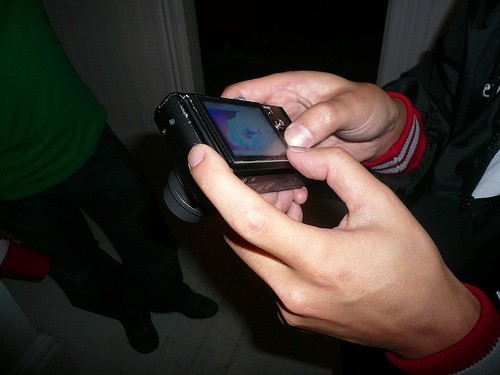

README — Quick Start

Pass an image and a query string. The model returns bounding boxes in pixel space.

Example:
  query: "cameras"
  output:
[154,91,313,223]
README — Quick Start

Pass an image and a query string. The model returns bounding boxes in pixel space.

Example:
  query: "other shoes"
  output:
[125,306,159,355]
[172,284,218,320]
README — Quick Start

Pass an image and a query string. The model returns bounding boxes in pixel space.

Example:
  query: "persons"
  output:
[0,0,217,356]
[187,0,500,375]
